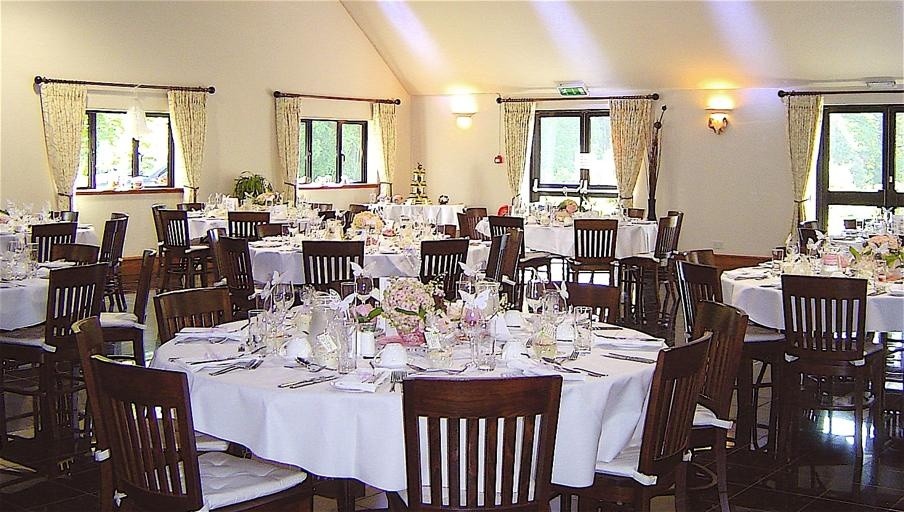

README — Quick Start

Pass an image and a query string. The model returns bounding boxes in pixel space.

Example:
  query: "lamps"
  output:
[705,107,734,133]
[452,112,474,129]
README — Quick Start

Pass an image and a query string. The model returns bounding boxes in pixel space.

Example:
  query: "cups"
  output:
[1,201,53,281]
[507,199,629,224]
[182,192,470,256]
[760,219,904,291]
[167,272,660,390]
